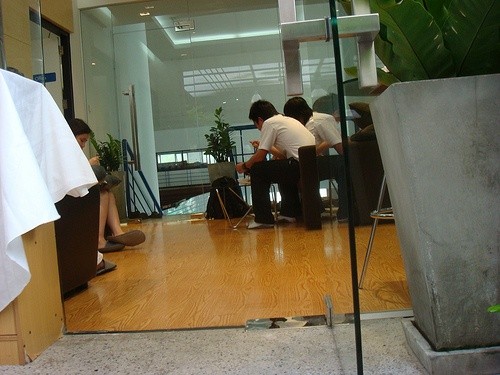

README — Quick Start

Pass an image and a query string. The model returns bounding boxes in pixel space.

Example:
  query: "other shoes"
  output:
[246,220,274,229]
[274,216,296,222]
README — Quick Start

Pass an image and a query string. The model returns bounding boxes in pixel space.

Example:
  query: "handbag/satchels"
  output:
[206,177,249,219]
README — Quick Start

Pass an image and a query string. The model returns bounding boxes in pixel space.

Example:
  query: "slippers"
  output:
[95,258,117,276]
[98,240,124,253]
[107,230,145,246]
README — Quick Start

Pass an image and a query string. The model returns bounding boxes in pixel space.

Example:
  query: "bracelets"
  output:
[242,161,250,172]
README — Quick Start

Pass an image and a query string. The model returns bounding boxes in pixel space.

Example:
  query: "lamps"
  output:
[250,88,261,103]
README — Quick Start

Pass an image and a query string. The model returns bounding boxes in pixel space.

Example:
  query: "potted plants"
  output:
[89,128,125,219]
[366,0,500,349]
[203,106,237,188]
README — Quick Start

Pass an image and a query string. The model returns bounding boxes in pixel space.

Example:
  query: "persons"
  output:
[235,94,360,229]
[67,119,146,275]
